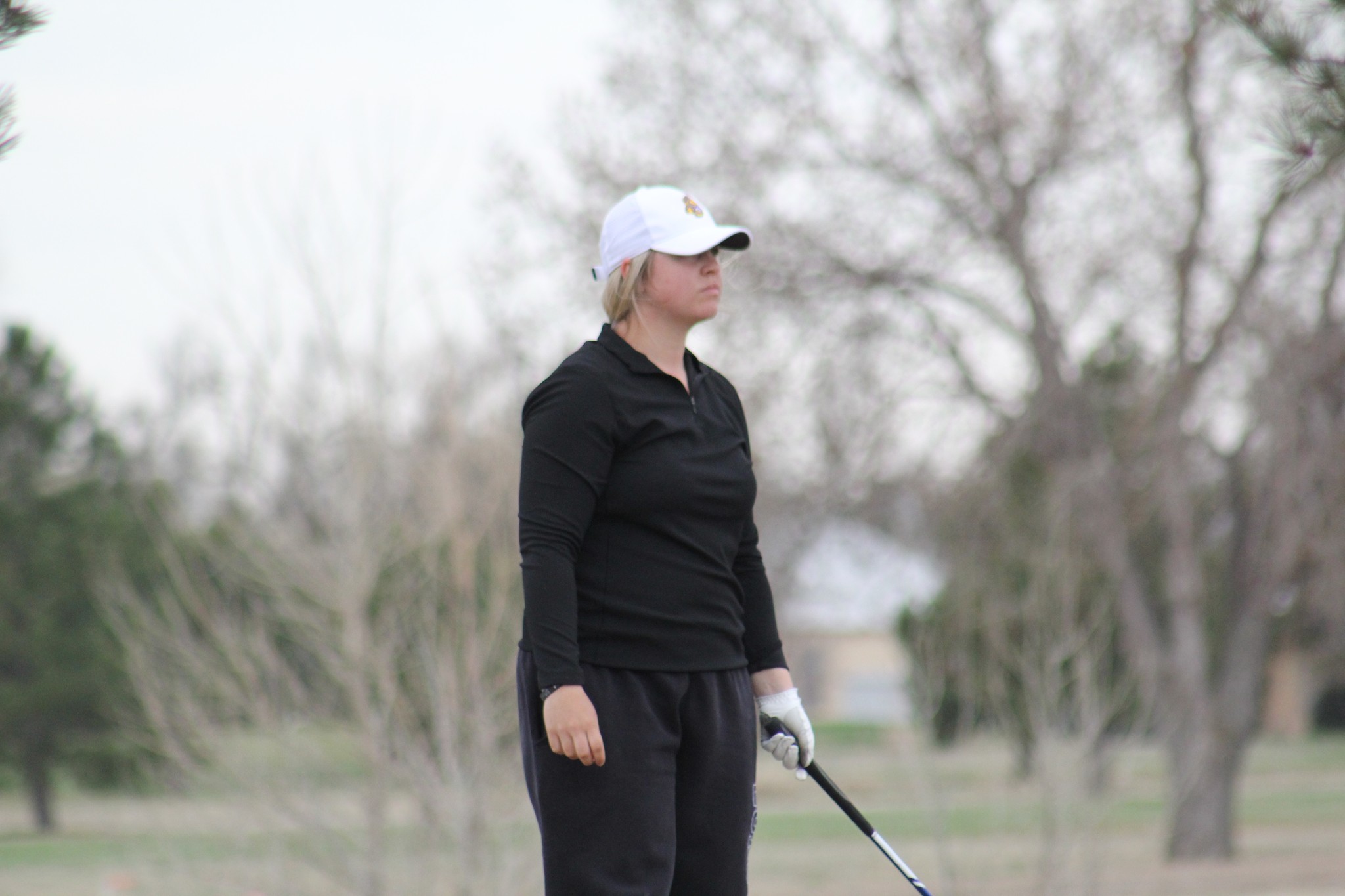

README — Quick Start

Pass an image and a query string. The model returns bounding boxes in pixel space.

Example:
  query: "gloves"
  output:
[756,688,815,779]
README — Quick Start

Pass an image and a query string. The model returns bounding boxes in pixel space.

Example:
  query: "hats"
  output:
[591,184,752,279]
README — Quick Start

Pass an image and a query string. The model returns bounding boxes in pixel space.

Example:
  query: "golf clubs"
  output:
[763,715,930,896]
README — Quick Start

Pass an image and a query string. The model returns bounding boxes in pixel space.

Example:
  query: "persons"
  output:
[516,186,815,896]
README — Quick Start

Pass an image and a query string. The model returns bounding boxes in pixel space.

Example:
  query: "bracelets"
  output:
[540,684,562,699]
